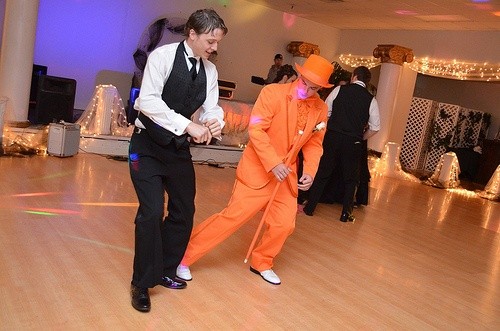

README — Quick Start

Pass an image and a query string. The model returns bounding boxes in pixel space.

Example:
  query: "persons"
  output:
[299,66,380,222]
[128,9,229,313]
[176,53,335,285]
[265,53,297,85]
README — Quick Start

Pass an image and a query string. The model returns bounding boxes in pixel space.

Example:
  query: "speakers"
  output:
[47,123,81,157]
[35,75,76,123]
[30,64,47,101]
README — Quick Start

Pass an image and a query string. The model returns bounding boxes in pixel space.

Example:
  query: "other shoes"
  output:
[250,265,281,286]
[303,206,314,216]
[340,212,356,223]
[176,264,193,281]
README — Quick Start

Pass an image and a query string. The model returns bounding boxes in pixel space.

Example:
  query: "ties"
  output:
[184,49,197,81]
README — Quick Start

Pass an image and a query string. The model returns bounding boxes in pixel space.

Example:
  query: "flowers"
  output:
[313,122,326,132]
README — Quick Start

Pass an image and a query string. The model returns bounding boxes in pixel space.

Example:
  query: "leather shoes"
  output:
[130,281,151,312]
[158,275,187,289]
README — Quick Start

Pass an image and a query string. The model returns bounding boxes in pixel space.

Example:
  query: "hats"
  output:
[275,54,283,59]
[295,54,334,88]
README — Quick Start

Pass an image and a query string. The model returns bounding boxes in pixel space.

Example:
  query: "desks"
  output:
[218,99,254,135]
